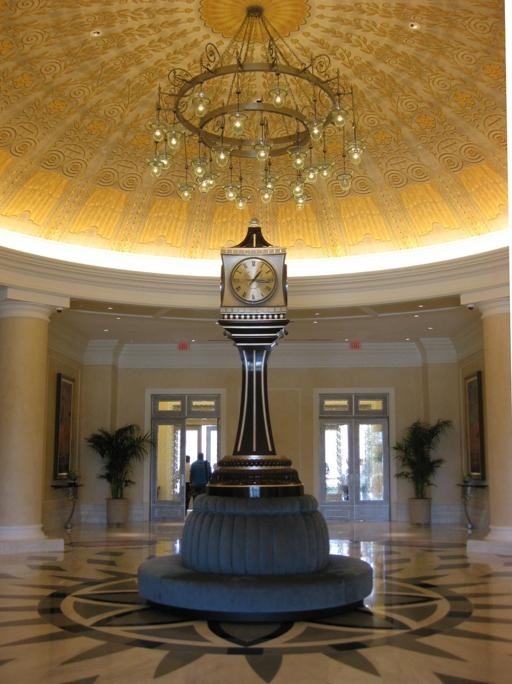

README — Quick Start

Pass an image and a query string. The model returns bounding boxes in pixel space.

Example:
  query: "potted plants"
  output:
[389,416,456,525]
[82,423,156,528]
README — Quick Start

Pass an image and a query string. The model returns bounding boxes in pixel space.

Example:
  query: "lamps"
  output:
[147,5,367,211]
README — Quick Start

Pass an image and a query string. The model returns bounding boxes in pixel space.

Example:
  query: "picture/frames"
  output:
[52,373,76,481]
[463,371,486,481]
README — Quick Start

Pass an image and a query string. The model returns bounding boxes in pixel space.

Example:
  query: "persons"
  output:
[184,455,194,517]
[189,453,212,500]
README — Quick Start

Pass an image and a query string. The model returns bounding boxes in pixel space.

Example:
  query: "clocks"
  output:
[204,215,304,498]
